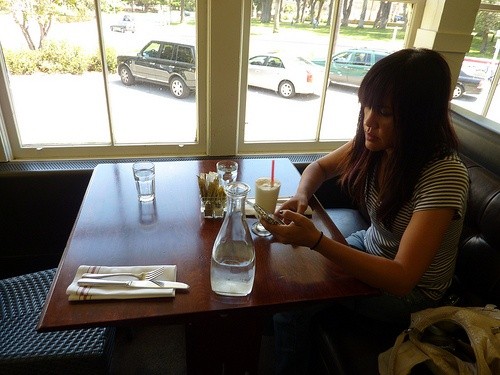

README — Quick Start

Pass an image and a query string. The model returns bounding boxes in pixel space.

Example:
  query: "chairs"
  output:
[0,269,115,375]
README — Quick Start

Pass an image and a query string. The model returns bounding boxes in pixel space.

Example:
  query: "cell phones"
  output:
[253,202,287,225]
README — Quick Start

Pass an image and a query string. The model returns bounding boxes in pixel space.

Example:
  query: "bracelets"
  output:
[310,231,323,249]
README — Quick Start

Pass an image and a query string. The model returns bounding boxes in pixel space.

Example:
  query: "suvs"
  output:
[116,38,197,99]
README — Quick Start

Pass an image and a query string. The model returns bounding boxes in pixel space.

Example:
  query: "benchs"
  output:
[320,153,500,375]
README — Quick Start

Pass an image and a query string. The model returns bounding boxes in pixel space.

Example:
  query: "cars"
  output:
[109,22,136,34]
[123,14,130,21]
[449,70,483,99]
[184,11,191,16]
[247,51,330,98]
[310,46,394,89]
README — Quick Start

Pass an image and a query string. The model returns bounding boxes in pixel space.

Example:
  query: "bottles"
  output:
[209,181,256,296]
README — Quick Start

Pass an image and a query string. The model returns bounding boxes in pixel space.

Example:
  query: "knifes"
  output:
[77,278,190,290]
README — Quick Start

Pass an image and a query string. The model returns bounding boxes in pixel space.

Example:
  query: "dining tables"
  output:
[35,157,385,375]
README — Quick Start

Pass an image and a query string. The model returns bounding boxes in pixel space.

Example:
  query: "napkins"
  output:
[66,265,178,301]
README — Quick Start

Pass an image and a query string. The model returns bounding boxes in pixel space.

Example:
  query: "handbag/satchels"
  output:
[387,305,500,375]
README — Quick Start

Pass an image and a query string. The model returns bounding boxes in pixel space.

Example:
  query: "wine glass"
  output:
[250,177,282,237]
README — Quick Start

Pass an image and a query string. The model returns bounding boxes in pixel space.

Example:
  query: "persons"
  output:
[260,48,470,375]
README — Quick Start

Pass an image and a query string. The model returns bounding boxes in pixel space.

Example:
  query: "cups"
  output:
[216,160,241,188]
[132,160,155,202]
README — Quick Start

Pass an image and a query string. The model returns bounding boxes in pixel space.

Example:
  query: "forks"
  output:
[82,267,168,281]
